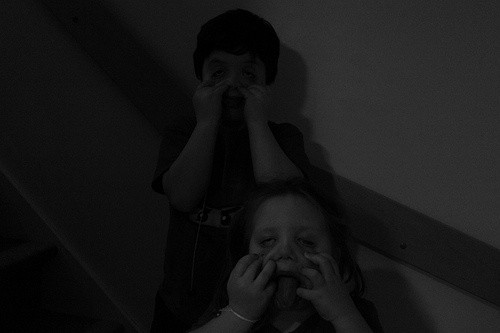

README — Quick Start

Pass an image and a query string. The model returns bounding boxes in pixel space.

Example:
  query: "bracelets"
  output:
[214,305,263,325]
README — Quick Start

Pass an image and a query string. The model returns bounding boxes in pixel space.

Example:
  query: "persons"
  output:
[146,5,313,333]
[184,176,386,333]
